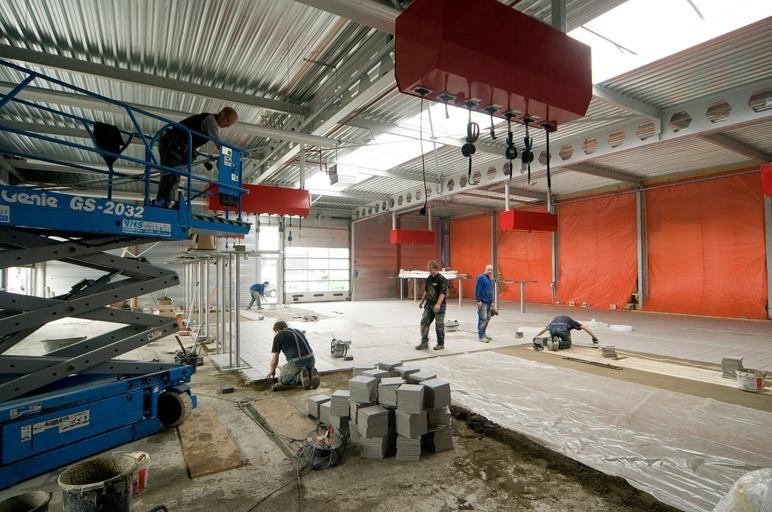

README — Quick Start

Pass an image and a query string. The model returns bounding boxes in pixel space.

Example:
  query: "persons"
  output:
[533,316,599,351]
[267,322,320,390]
[416,261,447,350]
[475,265,495,343]
[247,282,269,309]
[157,107,238,208]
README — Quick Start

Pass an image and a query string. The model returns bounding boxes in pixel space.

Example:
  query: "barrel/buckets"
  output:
[57,454,147,512]
[0,490,53,512]
[128,450,150,501]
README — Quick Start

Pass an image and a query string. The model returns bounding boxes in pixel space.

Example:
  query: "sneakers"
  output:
[434,344,444,350]
[546,336,559,351]
[311,368,320,389]
[481,336,492,343]
[416,343,428,349]
[300,368,311,389]
